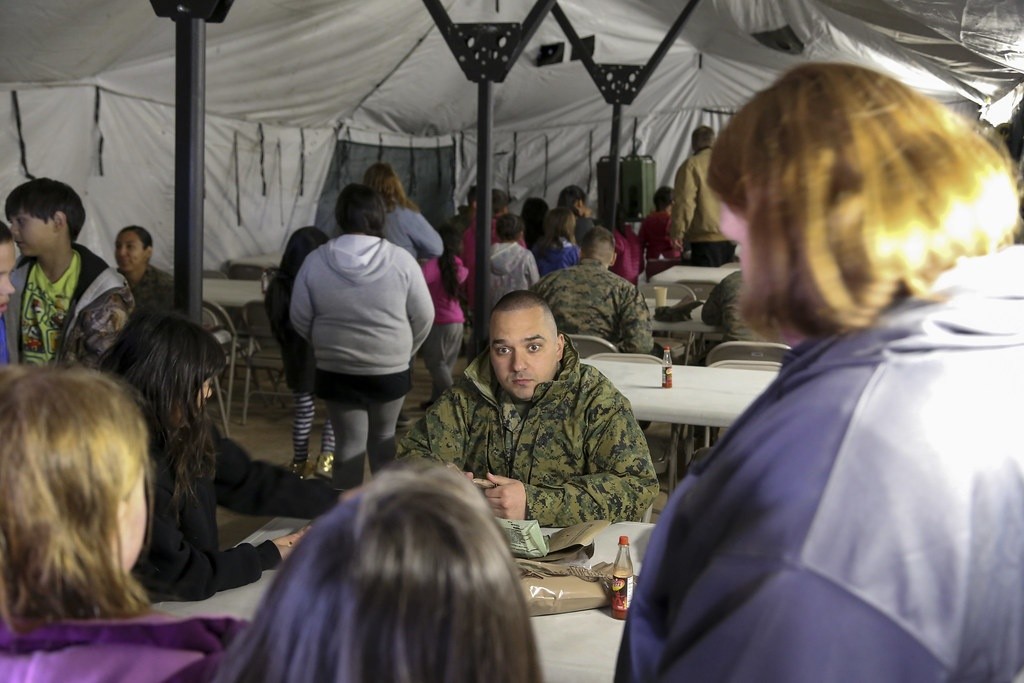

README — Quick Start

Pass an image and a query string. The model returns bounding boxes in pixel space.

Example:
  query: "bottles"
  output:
[611,536,633,618]
[662,346,672,388]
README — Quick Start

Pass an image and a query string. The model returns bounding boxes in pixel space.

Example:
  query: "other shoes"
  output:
[285,455,314,481]
[313,453,335,482]
[396,412,411,426]
[420,401,434,411]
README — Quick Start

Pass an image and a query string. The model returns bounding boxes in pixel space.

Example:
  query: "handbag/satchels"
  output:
[513,558,641,617]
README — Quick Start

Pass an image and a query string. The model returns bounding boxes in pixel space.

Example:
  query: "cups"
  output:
[653,287,667,307]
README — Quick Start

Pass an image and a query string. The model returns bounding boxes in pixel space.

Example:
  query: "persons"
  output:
[395,291,658,531]
[216,462,539,683]
[264,124,788,485]
[0,179,361,683]
[611,64,1024,683]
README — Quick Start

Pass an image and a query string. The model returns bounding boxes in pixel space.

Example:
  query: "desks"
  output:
[202,279,271,310]
[579,357,782,427]
[145,515,657,683]
[649,266,741,284]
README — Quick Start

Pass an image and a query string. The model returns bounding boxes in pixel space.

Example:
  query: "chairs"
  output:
[584,277,792,501]
[201,264,284,442]
